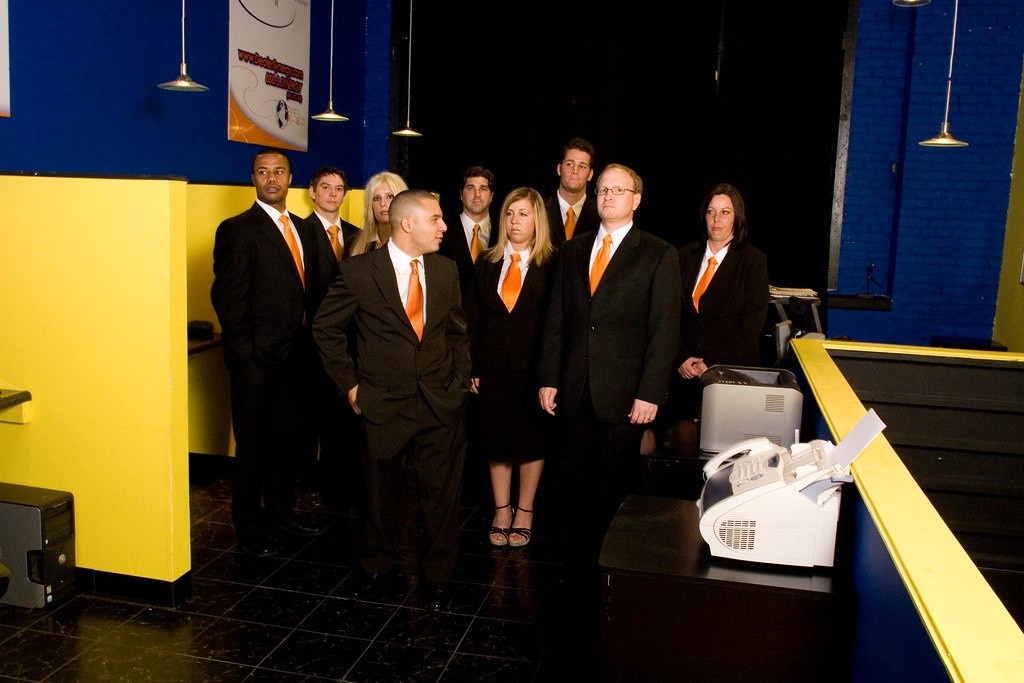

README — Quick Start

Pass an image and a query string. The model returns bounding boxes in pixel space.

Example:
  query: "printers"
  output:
[697,408,887,566]
[698,364,802,461]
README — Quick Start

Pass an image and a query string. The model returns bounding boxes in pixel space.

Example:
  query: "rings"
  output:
[644,417,650,420]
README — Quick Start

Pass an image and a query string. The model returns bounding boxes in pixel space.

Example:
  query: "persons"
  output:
[350,170,410,549]
[435,166,501,293]
[675,183,770,494]
[537,163,682,474]
[311,189,473,573]
[211,147,318,557]
[540,137,602,252]
[304,165,366,382]
[460,188,560,546]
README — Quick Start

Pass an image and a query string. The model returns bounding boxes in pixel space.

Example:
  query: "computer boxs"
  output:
[0,482,76,609]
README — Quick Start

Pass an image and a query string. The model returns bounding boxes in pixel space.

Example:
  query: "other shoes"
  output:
[240,533,276,557]
[292,506,329,532]
[426,581,450,613]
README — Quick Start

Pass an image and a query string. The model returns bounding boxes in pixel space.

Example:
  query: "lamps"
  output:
[311,0,349,122]
[392,0,423,136]
[918,0,969,147]
[157,0,210,92]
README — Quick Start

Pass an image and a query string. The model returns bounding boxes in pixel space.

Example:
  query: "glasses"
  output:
[595,186,638,195]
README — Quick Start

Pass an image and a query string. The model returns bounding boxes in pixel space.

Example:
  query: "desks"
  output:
[597,420,833,683]
[769,295,823,334]
[189,332,222,356]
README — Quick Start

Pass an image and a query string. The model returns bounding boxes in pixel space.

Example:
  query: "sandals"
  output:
[489,503,515,546]
[509,506,535,549]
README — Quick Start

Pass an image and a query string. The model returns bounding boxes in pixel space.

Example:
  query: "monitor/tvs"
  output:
[774,296,822,362]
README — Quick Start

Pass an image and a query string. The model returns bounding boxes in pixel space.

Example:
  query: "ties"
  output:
[327,226,344,264]
[501,255,522,314]
[470,224,482,265]
[589,235,613,297]
[278,215,307,324]
[564,207,578,241]
[404,259,424,343]
[692,257,718,313]
